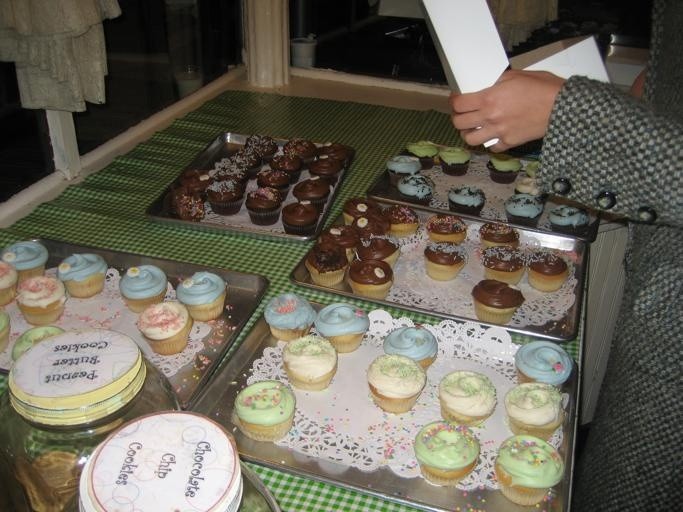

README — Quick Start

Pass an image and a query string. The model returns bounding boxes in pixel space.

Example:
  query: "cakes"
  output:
[227,379,297,444]
[514,338,575,389]
[304,196,570,326]
[1,240,228,363]
[502,380,565,441]
[493,433,566,509]
[385,139,592,236]
[413,418,483,487]
[163,132,348,237]
[439,369,497,432]
[263,291,440,416]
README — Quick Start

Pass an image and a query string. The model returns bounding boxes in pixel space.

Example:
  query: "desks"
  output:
[0,65,597,507]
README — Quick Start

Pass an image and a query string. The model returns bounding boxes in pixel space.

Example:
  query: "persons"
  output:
[448,2,680,512]
